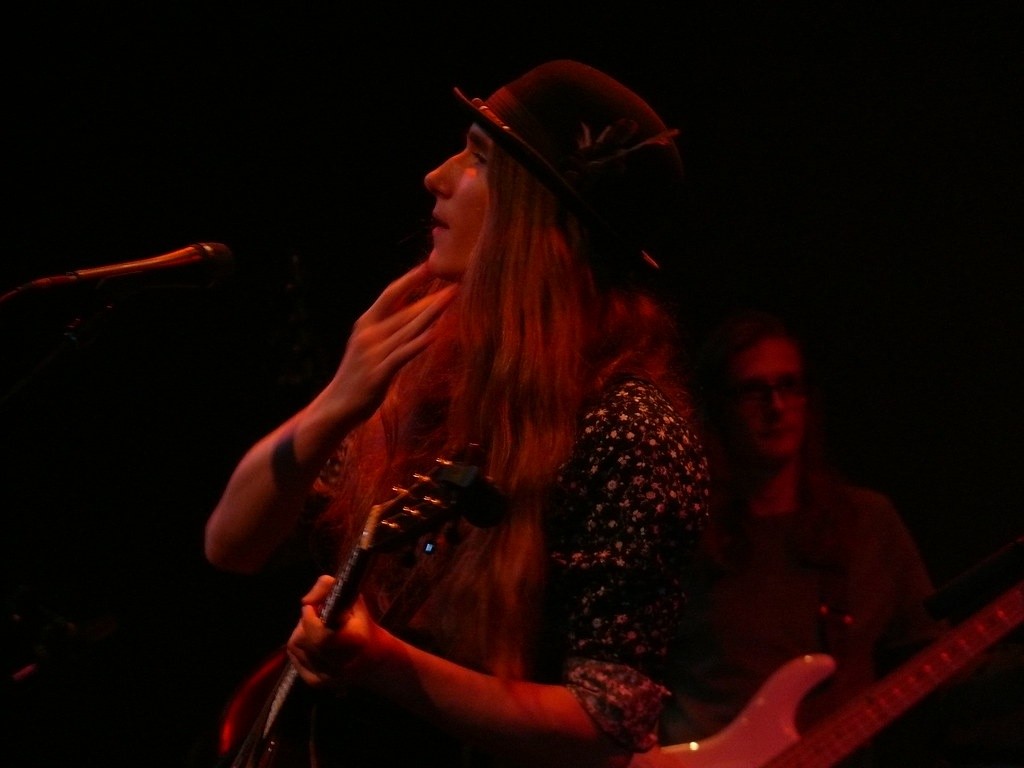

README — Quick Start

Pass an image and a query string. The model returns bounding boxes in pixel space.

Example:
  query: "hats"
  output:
[453,59,687,290]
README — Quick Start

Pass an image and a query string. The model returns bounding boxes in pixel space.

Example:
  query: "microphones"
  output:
[24,243,237,298]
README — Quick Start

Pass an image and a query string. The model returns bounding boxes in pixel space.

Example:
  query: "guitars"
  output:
[232,444,493,768]
[629,582,1023,766]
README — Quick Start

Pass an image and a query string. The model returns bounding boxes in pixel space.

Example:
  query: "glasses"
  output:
[709,368,819,408]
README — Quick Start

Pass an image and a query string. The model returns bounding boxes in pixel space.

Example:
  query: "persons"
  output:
[697,315,1001,763]
[206,61,707,768]
